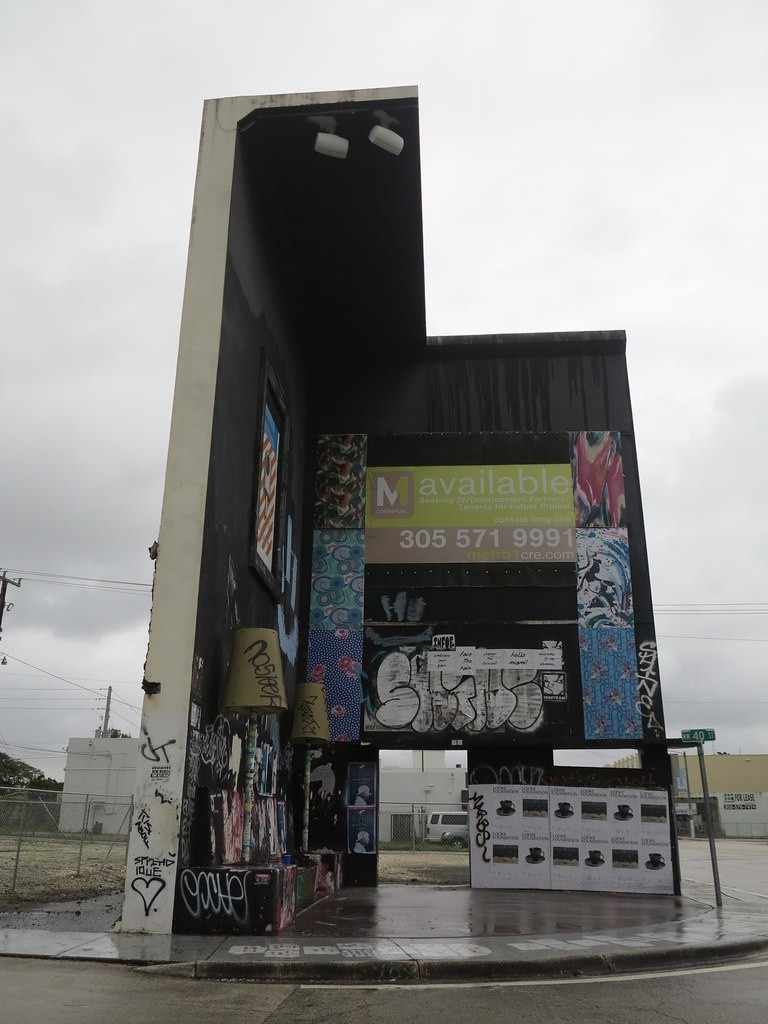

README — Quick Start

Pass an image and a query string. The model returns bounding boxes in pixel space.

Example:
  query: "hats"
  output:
[355,831,369,841]
[356,785,369,795]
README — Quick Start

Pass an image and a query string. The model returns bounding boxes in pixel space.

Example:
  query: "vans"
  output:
[424,811,469,849]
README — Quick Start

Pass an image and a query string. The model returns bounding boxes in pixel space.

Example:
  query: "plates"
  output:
[613,812,634,821]
[496,807,516,817]
[584,857,605,868]
[554,810,575,818]
[617,804,633,818]
[525,855,546,864]
[644,861,666,870]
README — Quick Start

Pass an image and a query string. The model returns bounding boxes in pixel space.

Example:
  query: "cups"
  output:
[648,853,665,867]
[500,800,515,813]
[528,847,545,861]
[558,802,573,815]
[588,849,604,865]
[649,853,664,866]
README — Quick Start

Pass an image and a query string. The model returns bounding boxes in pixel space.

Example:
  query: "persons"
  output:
[355,785,370,806]
[354,831,369,853]
